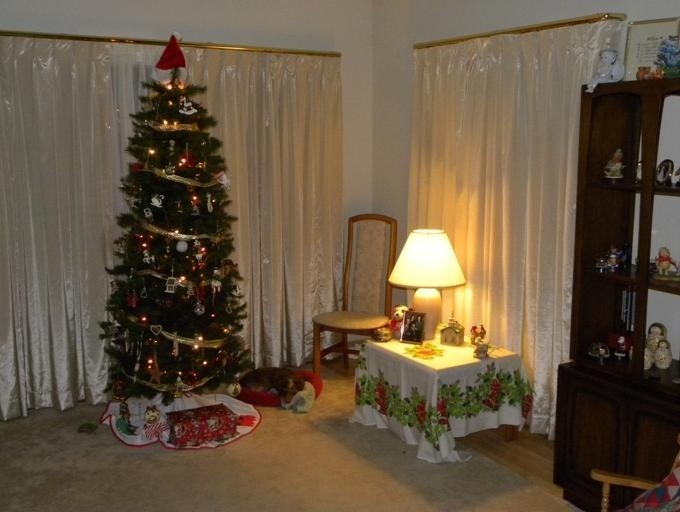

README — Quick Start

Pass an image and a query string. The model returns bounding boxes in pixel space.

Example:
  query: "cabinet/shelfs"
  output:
[551,76,680,404]
[550,359,680,512]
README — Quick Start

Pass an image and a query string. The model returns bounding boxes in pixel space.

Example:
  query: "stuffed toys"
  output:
[384,305,414,343]
[655,247,679,276]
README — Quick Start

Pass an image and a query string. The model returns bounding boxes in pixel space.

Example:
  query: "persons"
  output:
[404,313,423,342]
[584,43,618,93]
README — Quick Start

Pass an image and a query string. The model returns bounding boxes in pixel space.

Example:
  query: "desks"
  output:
[347,336,535,464]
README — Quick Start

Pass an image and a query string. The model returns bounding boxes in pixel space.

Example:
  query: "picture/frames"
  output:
[623,17,679,79]
[401,309,427,345]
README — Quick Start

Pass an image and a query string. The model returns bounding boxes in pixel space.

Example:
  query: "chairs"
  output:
[312,212,398,379]
[588,431,680,512]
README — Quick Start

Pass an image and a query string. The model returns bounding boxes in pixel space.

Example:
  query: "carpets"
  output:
[0,358,587,511]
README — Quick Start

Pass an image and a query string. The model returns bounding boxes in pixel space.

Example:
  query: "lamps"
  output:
[387,228,468,342]
